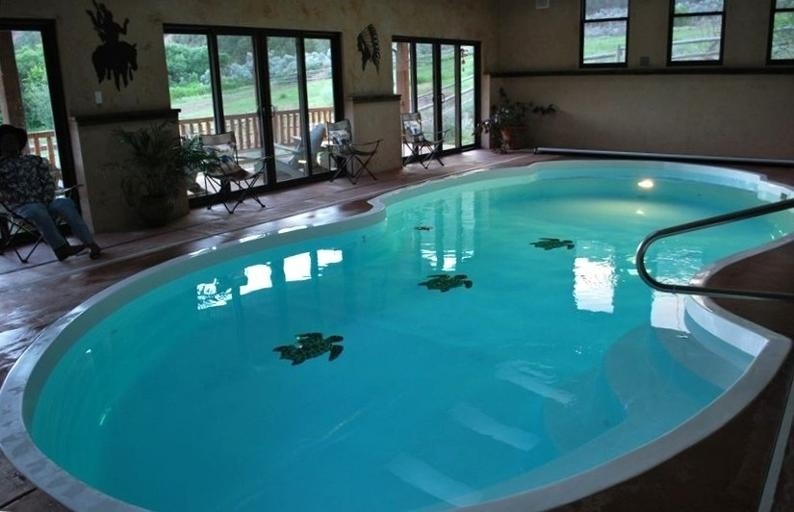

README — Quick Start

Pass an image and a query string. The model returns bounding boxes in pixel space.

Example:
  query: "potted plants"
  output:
[107,115,231,229]
[472,83,559,149]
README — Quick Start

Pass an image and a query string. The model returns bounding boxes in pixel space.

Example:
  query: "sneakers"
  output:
[54,242,88,262]
[84,241,101,260]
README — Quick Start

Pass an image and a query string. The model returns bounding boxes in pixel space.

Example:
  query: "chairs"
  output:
[253,117,384,186]
[200,130,269,214]
[0,160,85,265]
[399,111,450,171]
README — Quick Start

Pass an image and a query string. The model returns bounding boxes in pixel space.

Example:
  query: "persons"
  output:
[0,124,101,261]
